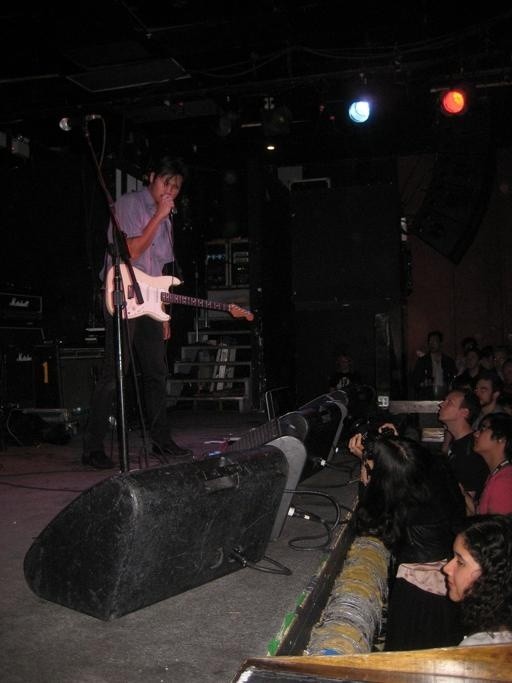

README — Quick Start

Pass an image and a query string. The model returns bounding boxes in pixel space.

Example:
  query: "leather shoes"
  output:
[152,440,192,459]
[81,452,115,469]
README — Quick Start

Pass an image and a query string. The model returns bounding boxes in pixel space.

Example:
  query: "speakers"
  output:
[24,444,289,622]
[229,389,349,539]
[40,343,115,422]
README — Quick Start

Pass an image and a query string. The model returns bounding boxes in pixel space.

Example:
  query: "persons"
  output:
[328,332,511,652]
[82,148,193,470]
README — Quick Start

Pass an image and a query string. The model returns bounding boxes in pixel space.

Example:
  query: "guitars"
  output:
[105,264,254,322]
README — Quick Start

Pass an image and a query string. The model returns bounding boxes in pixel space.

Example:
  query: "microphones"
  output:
[59,114,98,132]
[168,203,177,216]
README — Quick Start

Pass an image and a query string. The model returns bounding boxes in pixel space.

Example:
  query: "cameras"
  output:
[351,419,395,455]
